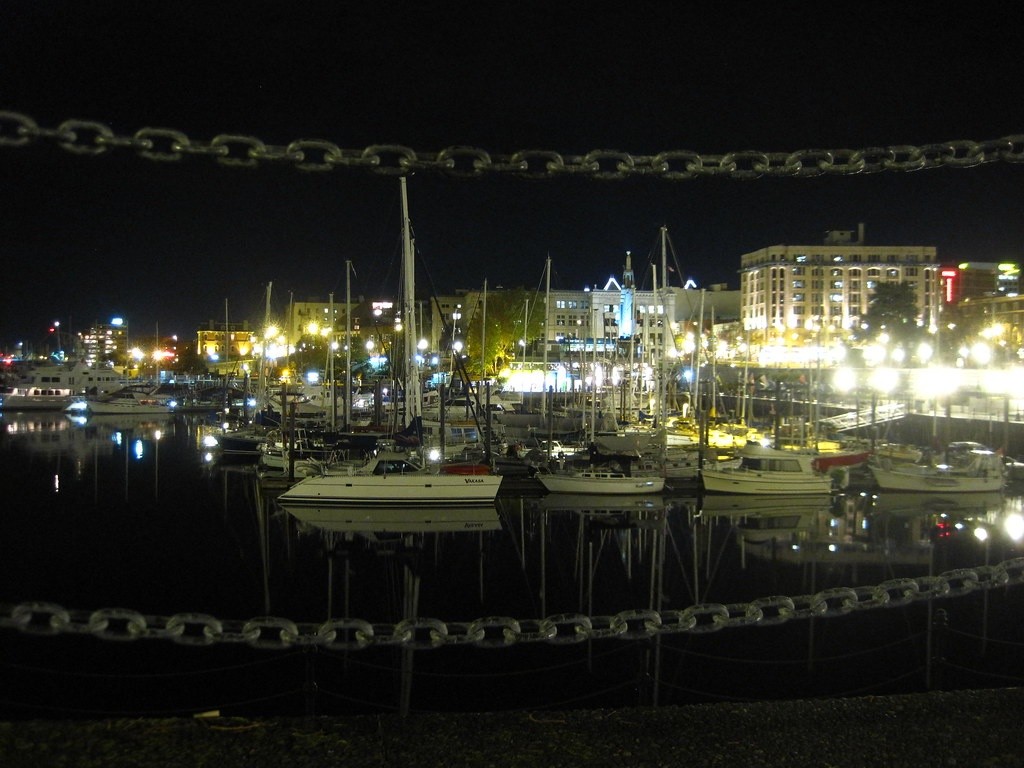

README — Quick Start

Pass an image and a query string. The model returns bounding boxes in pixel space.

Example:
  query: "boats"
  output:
[700,443,834,497]
[2,321,128,410]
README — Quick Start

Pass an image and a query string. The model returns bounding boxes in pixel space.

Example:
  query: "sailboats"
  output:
[512,221,706,497]
[217,261,507,478]
[277,504,504,715]
[86,321,175,415]
[276,174,506,502]
[864,258,1024,493]
[524,492,842,710]
[516,252,870,480]
